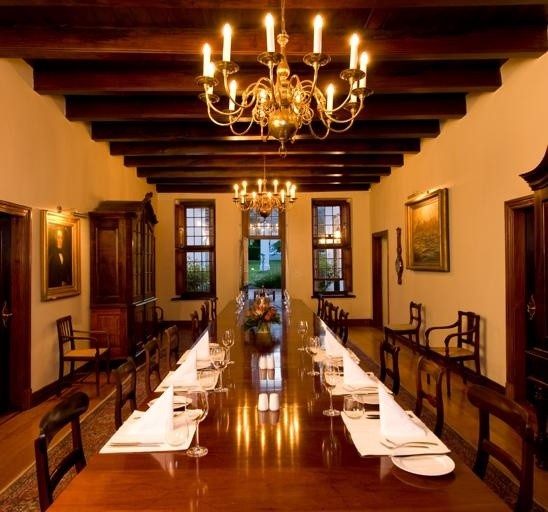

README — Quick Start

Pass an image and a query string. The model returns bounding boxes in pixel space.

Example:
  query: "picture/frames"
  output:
[40,208,81,301]
[404,188,450,272]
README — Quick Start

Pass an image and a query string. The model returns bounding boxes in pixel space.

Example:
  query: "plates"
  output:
[391,454,457,476]
[149,395,190,409]
[355,392,391,404]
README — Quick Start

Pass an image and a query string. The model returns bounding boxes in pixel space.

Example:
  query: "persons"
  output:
[49,227,68,288]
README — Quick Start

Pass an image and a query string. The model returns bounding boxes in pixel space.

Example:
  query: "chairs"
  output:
[34,393,89,512]
[467,385,538,512]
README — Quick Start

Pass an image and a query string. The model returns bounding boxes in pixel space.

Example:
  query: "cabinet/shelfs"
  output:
[89,192,158,359]
[503,145,548,470]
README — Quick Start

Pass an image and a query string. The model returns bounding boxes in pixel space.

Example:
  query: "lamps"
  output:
[193,0,374,158]
[232,156,299,223]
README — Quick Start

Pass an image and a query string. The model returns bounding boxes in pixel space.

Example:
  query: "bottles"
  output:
[258,393,267,413]
[268,394,280,414]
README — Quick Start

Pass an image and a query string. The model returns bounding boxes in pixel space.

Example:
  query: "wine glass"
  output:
[186,455,208,511]
[184,288,365,459]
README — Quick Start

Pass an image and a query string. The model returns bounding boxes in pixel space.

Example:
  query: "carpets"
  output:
[0,334,548,512]
